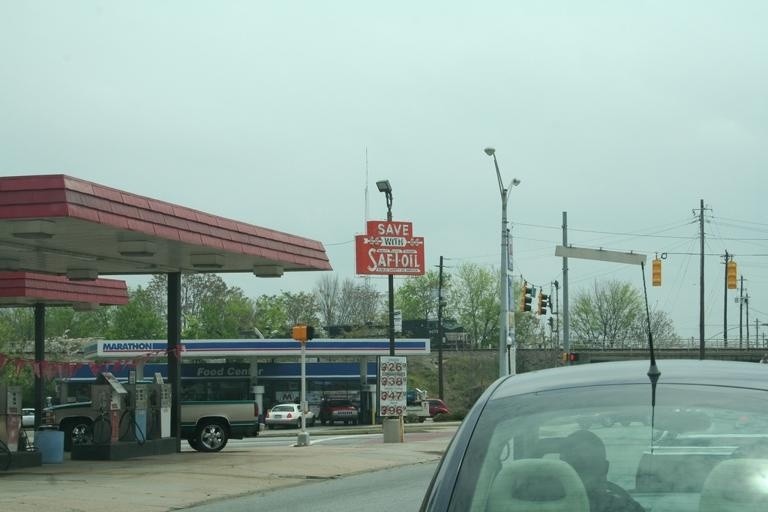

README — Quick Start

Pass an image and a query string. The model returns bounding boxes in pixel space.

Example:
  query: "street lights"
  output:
[484,147,521,379]
[375,180,405,442]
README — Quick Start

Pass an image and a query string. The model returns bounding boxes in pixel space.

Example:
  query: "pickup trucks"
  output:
[38,380,260,453]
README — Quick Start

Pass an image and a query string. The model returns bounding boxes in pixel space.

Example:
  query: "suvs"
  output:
[424,398,450,421]
[318,400,359,425]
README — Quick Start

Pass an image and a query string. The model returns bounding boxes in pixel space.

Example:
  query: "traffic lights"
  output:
[293,325,315,341]
[546,316,553,327]
[546,294,553,308]
[570,353,579,360]
[518,284,533,313]
[537,292,547,315]
[562,352,569,362]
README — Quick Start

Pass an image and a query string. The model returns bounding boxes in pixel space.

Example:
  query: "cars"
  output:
[265,402,316,430]
[419,261,768,511]
[575,404,651,428]
[20,407,35,428]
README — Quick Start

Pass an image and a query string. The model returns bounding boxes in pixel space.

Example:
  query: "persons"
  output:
[51,394,61,406]
[559,429,645,511]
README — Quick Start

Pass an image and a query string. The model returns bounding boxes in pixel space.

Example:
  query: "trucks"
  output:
[403,388,430,422]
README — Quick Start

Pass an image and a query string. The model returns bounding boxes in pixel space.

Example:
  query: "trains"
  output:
[183,317,472,351]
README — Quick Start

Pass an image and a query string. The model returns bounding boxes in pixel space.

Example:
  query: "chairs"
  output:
[486,458,589,511]
[697,456,766,511]
